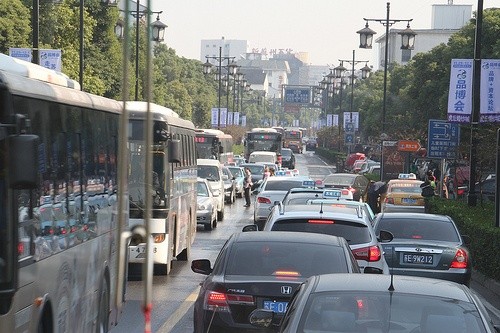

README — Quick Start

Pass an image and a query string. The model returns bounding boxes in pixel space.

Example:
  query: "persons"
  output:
[243,167,252,207]
[205,169,216,178]
[417,162,448,201]
[263,166,275,179]
[127,155,159,205]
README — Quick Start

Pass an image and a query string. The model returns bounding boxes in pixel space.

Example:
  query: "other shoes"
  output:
[243,204,250,207]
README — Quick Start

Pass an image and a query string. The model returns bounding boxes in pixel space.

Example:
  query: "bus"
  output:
[194,128,234,163]
[273,126,303,154]
[242,128,282,165]
[117,101,197,275]
[0,52,129,333]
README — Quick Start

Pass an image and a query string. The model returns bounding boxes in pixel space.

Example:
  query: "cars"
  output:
[15,179,117,260]
[352,160,381,175]
[281,148,296,168]
[251,173,375,231]
[371,212,472,284]
[302,137,319,151]
[377,179,425,213]
[248,271,500,333]
[191,231,383,333]
[181,151,280,231]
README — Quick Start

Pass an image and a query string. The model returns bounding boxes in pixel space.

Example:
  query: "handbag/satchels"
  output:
[249,182,253,186]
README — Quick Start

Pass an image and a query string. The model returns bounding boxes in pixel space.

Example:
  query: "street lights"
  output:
[119,11,169,101]
[201,56,250,129]
[356,18,417,181]
[317,60,372,154]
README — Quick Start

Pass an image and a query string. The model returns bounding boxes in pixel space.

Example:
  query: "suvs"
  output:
[241,201,393,276]
